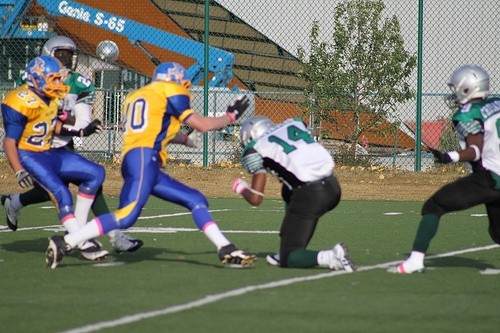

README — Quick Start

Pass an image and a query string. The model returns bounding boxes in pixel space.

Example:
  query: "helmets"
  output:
[41,36,78,71]
[238,116,275,149]
[152,62,191,89]
[26,56,70,99]
[444,65,489,108]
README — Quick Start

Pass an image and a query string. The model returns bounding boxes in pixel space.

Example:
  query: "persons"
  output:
[0,35,144,254]
[232,115,356,271]
[386,64,500,273]
[0,56,110,262]
[44,61,257,270]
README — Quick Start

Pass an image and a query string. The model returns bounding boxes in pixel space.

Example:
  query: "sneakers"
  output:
[386,262,425,273]
[266,253,280,265]
[78,239,101,251]
[329,242,357,272]
[81,249,109,261]
[110,234,144,254]
[218,247,258,266]
[45,235,72,269]
[0,194,19,231]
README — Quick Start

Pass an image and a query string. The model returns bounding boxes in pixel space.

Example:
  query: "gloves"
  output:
[232,178,248,195]
[428,146,460,165]
[81,119,105,137]
[186,133,200,147]
[227,95,250,121]
[15,169,34,189]
